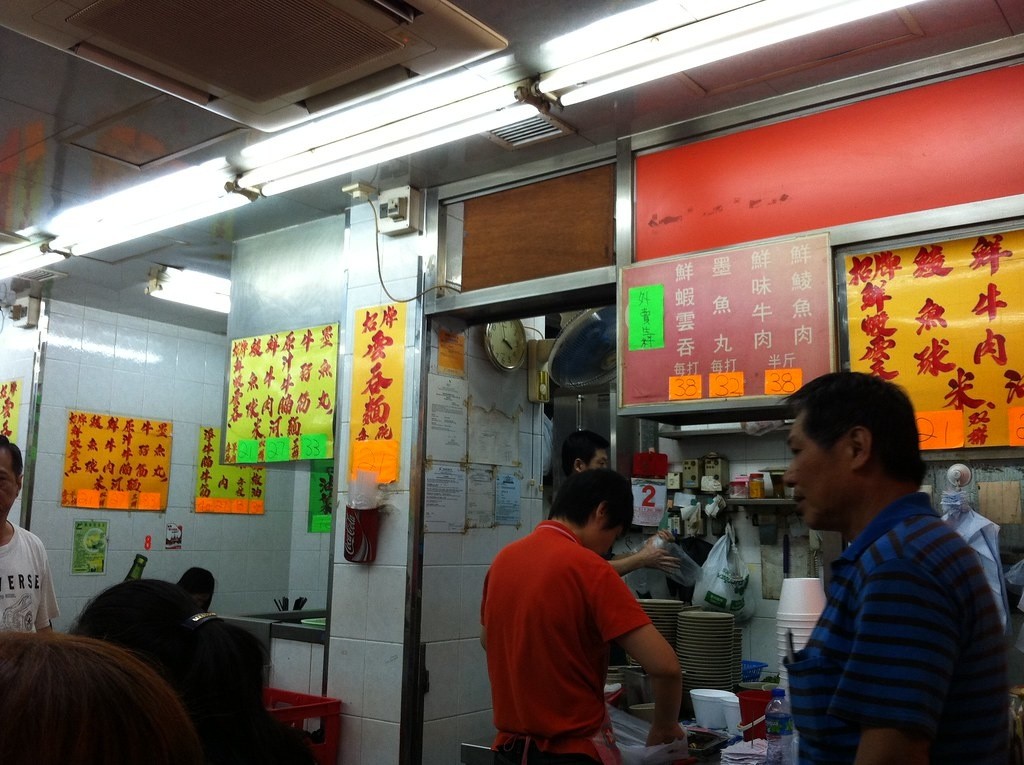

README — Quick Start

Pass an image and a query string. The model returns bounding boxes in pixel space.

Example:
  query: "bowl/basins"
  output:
[723,696,744,738]
[777,575,828,700]
[690,688,738,728]
[628,704,659,722]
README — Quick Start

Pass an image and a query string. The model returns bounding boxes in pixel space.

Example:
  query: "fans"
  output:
[525,304,618,492]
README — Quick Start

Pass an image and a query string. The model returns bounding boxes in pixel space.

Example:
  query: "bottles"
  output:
[729,479,750,499]
[120,554,148,584]
[769,469,786,500]
[749,472,764,499]
[765,687,793,765]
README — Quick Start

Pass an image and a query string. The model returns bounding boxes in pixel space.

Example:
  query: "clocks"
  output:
[483,316,529,374]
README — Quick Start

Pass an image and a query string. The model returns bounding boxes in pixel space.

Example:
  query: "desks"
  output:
[459,701,793,764]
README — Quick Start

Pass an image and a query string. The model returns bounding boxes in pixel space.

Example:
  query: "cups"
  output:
[344,503,383,564]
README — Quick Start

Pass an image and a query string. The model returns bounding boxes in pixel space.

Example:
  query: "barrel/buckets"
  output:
[736,691,781,742]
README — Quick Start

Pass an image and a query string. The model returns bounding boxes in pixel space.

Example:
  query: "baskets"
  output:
[741,659,769,682]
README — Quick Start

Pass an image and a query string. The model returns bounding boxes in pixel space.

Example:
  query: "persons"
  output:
[0,434,62,635]
[772,372,1013,765]
[0,567,320,765]
[478,468,686,765]
[548,430,682,578]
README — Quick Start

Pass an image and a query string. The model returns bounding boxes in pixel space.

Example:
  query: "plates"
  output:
[761,682,779,691]
[629,597,745,699]
[738,681,769,691]
[605,667,625,687]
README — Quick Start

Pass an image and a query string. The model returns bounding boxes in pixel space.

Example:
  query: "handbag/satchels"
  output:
[690,524,755,623]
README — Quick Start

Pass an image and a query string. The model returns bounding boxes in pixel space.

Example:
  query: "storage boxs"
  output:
[265,686,344,763]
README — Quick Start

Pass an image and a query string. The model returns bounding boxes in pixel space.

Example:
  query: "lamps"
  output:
[1,2,932,284]
